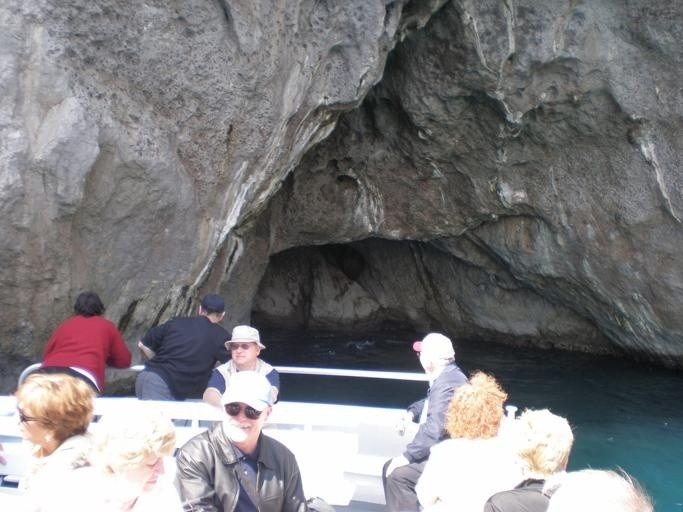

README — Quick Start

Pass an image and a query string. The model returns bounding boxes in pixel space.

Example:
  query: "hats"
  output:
[220,370,272,411]
[223,324,266,350]
[413,331,455,360]
[201,294,226,314]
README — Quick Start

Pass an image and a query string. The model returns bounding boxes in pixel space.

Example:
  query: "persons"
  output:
[75,398,183,512]
[38,291,132,398]
[483,408,574,512]
[542,466,655,512]
[203,325,280,408]
[15,372,100,512]
[134,294,232,401]
[415,370,517,512]
[382,332,469,512]
[175,371,307,512]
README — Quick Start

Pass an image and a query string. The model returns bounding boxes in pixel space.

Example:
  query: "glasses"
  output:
[16,408,44,423]
[221,400,263,420]
[228,342,257,350]
